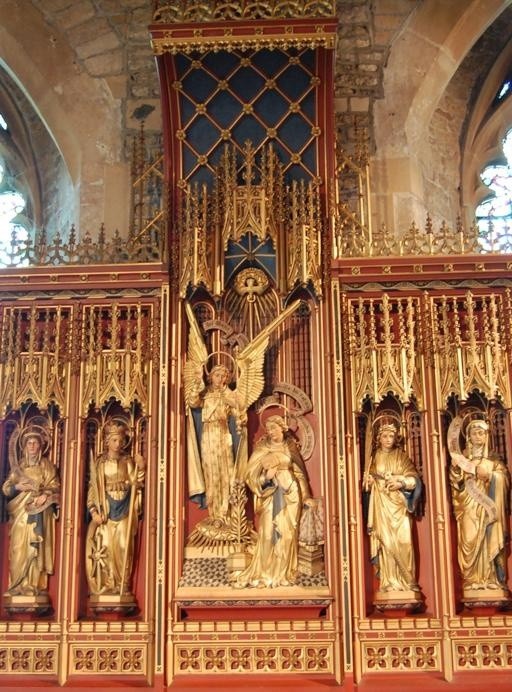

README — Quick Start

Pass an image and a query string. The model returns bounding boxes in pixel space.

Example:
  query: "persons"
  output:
[192,363,246,524]
[449,420,505,589]
[362,423,425,592]
[227,414,312,585]
[82,421,143,596]
[1,430,60,602]
